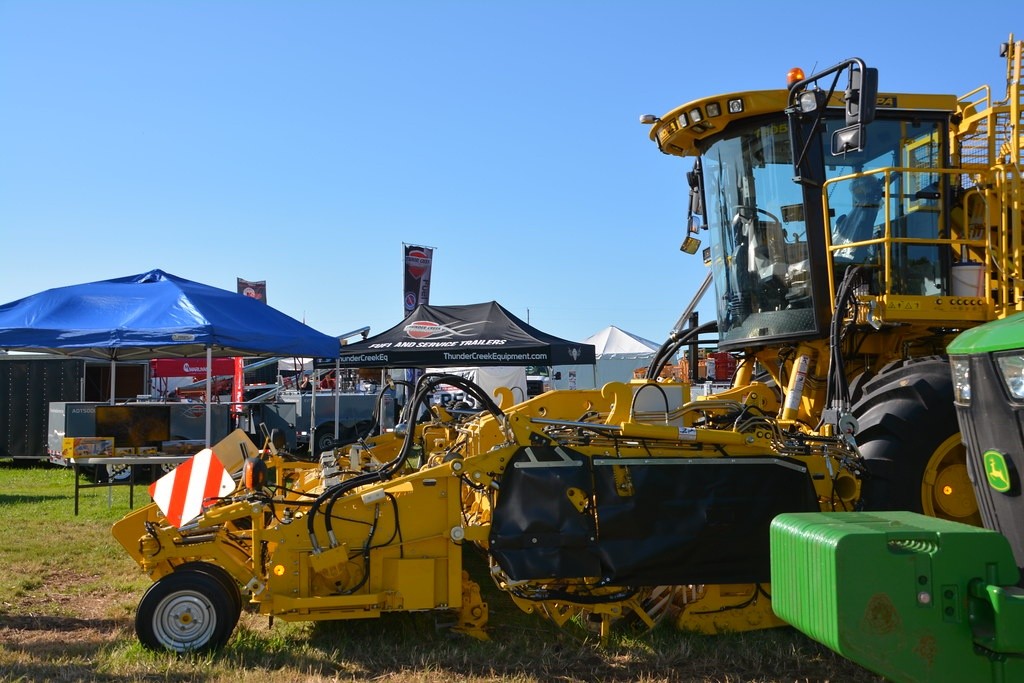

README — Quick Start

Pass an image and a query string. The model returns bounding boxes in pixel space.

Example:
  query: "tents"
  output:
[0,269,340,465]
[310,301,679,457]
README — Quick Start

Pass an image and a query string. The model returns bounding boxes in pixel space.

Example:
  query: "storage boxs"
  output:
[63,437,114,458]
[137,447,157,455]
[114,447,136,457]
[162,439,206,455]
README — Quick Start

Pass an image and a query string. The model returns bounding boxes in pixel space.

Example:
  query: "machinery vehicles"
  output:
[767,310,1024,683]
[109,33,1024,661]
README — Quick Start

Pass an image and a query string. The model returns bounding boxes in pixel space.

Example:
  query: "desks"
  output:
[71,455,194,516]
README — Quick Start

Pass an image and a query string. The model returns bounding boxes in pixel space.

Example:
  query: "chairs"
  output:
[788,175,883,284]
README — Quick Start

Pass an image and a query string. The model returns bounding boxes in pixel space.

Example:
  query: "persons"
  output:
[300,374,312,394]
[284,377,294,389]
[320,375,336,393]
